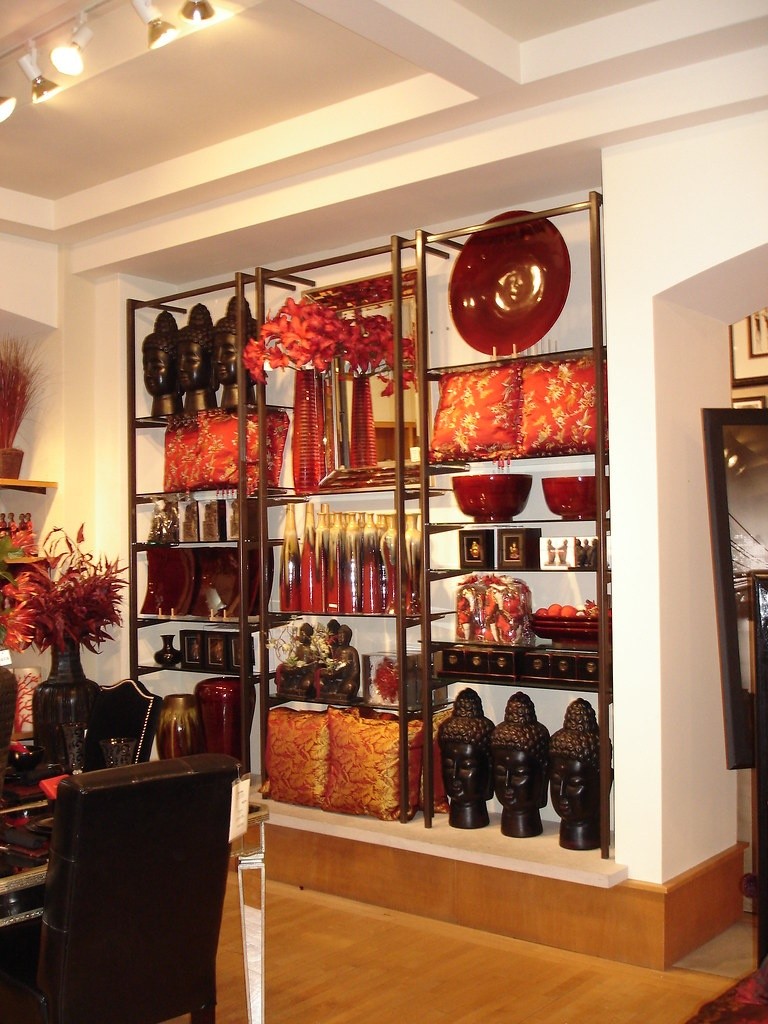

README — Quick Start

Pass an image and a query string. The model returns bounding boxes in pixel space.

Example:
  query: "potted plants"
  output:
[0,332,59,479]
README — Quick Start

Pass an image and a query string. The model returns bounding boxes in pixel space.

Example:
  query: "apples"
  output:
[536,604,578,617]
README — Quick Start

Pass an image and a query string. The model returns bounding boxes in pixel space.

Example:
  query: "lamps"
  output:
[0,96,16,123]
[17,39,61,104]
[50,12,94,75]
[130,0,177,49]
[181,0,214,21]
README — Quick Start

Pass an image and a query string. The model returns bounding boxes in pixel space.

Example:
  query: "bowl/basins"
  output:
[452,474,533,522]
[542,476,610,520]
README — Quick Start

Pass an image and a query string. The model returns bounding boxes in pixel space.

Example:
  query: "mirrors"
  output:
[701,407,768,772]
[301,265,434,494]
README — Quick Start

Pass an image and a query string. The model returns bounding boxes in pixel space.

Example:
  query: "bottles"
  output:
[155,635,180,665]
[279,503,423,614]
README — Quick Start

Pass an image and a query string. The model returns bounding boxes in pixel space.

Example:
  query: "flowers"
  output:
[241,296,364,387]
[345,307,418,398]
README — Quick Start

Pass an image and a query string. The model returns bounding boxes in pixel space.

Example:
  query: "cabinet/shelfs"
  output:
[0,478,58,563]
[126,191,616,861]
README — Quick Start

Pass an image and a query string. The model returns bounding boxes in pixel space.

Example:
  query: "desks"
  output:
[0,798,271,1024]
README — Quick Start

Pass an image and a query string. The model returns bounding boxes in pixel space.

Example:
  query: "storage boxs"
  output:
[361,647,448,710]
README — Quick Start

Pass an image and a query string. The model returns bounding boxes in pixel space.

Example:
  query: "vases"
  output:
[294,370,325,494]
[280,503,423,615]
[350,377,377,467]
[155,636,180,667]
[0,666,17,798]
[32,638,99,762]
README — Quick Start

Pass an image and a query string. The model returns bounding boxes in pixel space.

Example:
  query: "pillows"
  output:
[163,409,291,497]
[428,354,609,469]
[257,706,453,821]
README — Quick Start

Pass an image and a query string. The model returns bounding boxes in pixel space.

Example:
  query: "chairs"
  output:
[84,679,163,774]
[1,754,241,1024]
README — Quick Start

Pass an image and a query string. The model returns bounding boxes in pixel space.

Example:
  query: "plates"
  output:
[530,614,612,637]
[448,210,572,355]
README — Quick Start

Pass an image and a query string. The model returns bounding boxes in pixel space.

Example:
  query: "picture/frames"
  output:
[728,306,768,389]
[732,395,765,410]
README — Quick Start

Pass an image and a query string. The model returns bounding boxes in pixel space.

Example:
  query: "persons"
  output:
[437,687,614,851]
[275,619,360,696]
[142,296,257,417]
